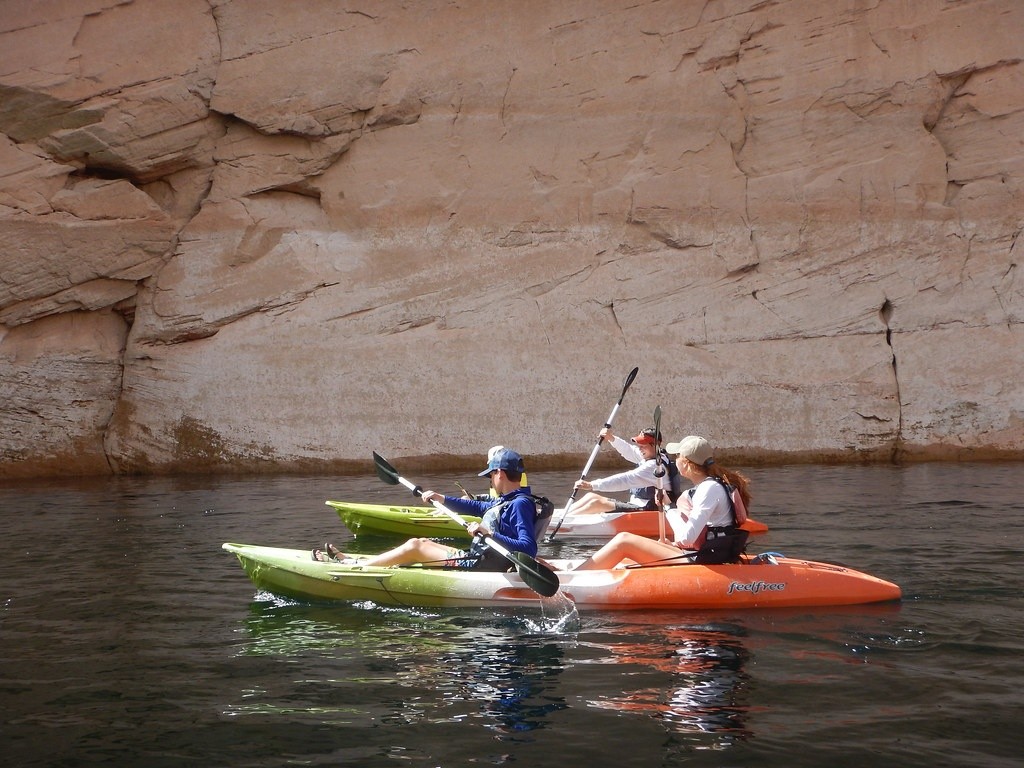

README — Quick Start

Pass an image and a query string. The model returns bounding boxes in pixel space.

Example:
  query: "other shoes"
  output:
[311,542,340,562]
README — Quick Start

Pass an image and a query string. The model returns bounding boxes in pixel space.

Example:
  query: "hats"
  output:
[631,427,662,445]
[486,445,504,464]
[478,449,525,476]
[666,436,714,465]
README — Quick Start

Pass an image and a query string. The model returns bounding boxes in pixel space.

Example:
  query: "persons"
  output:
[312,447,536,573]
[458,444,533,503]
[566,426,680,514]
[536,434,753,572]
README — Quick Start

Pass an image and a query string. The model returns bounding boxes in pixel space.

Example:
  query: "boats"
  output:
[219,542,904,612]
[326,500,771,542]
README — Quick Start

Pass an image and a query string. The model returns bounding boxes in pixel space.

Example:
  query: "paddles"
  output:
[372,449,560,599]
[652,404,670,543]
[546,365,640,542]
